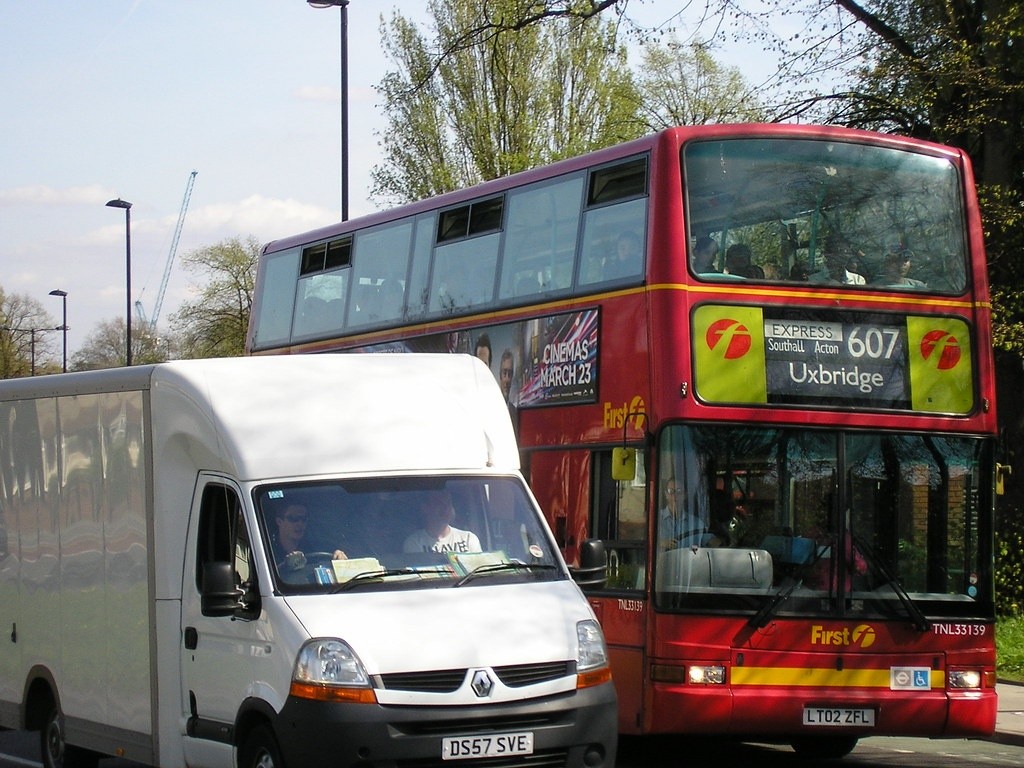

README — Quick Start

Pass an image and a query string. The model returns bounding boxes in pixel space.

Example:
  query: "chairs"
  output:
[235,507,251,581]
[300,276,539,333]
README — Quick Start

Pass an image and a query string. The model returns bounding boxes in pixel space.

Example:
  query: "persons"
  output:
[656,476,722,554]
[403,484,483,553]
[801,489,868,592]
[474,335,518,442]
[297,231,927,335]
[250,495,349,573]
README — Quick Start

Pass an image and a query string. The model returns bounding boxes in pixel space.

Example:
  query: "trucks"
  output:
[1,352,620,767]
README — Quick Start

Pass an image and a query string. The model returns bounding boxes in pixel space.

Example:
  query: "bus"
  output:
[243,123,1013,763]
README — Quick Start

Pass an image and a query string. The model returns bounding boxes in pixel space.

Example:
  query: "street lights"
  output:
[48,289,68,373]
[1,325,71,377]
[307,0,350,221]
[105,200,132,365]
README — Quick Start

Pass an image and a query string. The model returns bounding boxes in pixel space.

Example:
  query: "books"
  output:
[313,550,523,584]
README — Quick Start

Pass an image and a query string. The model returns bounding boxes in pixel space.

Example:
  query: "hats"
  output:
[884,243,915,258]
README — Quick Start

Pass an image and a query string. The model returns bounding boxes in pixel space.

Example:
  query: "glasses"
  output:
[666,487,682,495]
[281,515,309,522]
